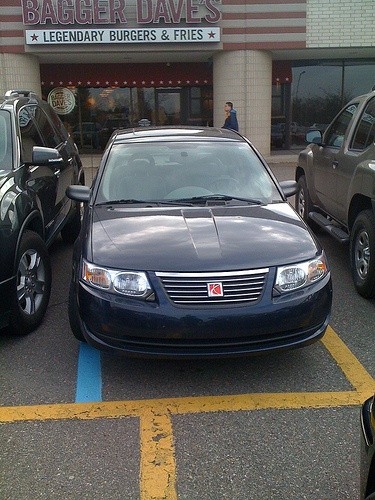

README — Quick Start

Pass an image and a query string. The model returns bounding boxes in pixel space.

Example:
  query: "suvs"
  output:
[0,88,85,335]
[294,86,375,300]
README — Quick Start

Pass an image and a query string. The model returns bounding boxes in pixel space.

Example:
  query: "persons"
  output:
[221,112,230,128]
[224,102,238,132]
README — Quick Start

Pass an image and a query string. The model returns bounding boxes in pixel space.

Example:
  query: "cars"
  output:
[271,125,284,149]
[72,122,100,146]
[65,125,334,362]
